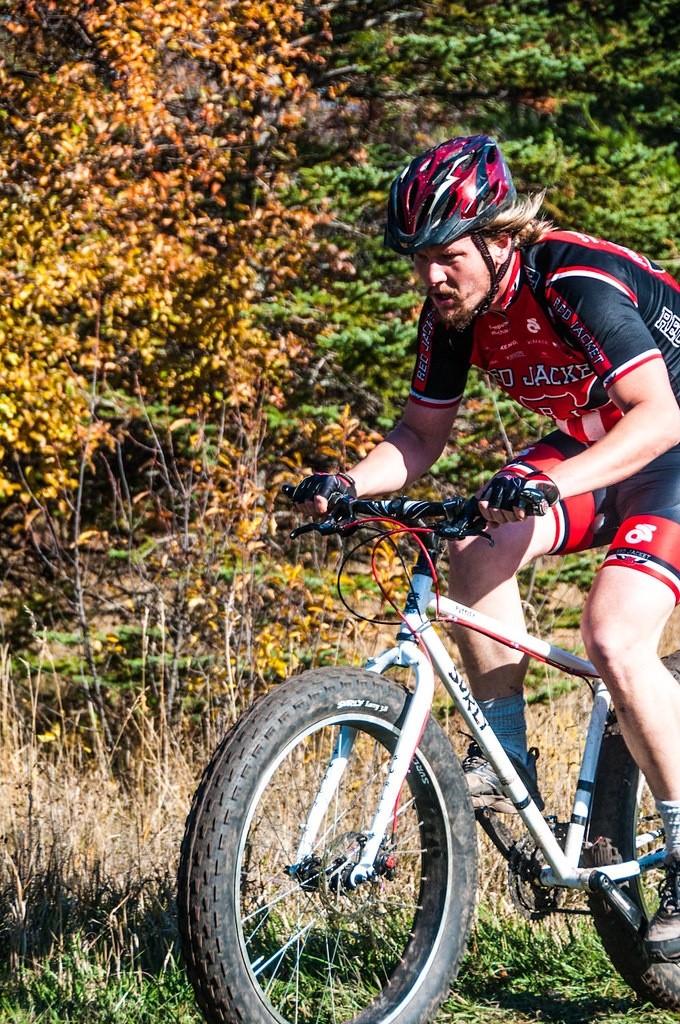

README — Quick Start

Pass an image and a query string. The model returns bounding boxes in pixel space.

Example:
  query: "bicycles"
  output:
[172,466,680,1023]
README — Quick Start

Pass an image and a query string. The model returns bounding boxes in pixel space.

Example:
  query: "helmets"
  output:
[383,134,518,255]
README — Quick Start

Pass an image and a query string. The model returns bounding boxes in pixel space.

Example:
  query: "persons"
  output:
[291,134,679,966]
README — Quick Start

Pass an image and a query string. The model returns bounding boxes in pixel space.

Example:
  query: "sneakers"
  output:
[642,847,680,964]
[460,731,545,813]
